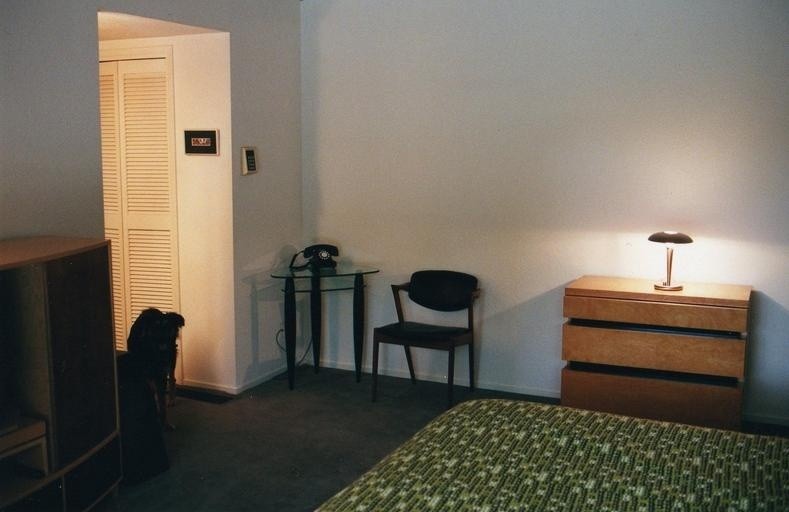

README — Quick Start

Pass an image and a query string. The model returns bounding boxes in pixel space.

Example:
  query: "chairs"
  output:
[371,271,477,403]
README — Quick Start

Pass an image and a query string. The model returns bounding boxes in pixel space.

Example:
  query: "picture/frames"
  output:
[183,128,222,156]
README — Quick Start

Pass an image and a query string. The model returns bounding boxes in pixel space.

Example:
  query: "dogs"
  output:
[126,307,185,433]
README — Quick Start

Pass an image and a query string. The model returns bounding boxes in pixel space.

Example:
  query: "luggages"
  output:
[118,363,171,486]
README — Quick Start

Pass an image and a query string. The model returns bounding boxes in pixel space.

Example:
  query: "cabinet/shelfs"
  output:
[0,235,125,512]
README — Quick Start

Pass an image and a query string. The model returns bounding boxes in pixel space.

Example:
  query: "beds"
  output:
[313,397,788,510]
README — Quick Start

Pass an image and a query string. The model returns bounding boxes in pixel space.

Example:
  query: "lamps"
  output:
[648,230,695,292]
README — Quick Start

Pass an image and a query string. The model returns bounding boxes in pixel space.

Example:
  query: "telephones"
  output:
[304,244,339,268]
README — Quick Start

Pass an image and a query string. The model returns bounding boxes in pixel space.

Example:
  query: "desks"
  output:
[271,263,380,390]
[559,275,752,433]
